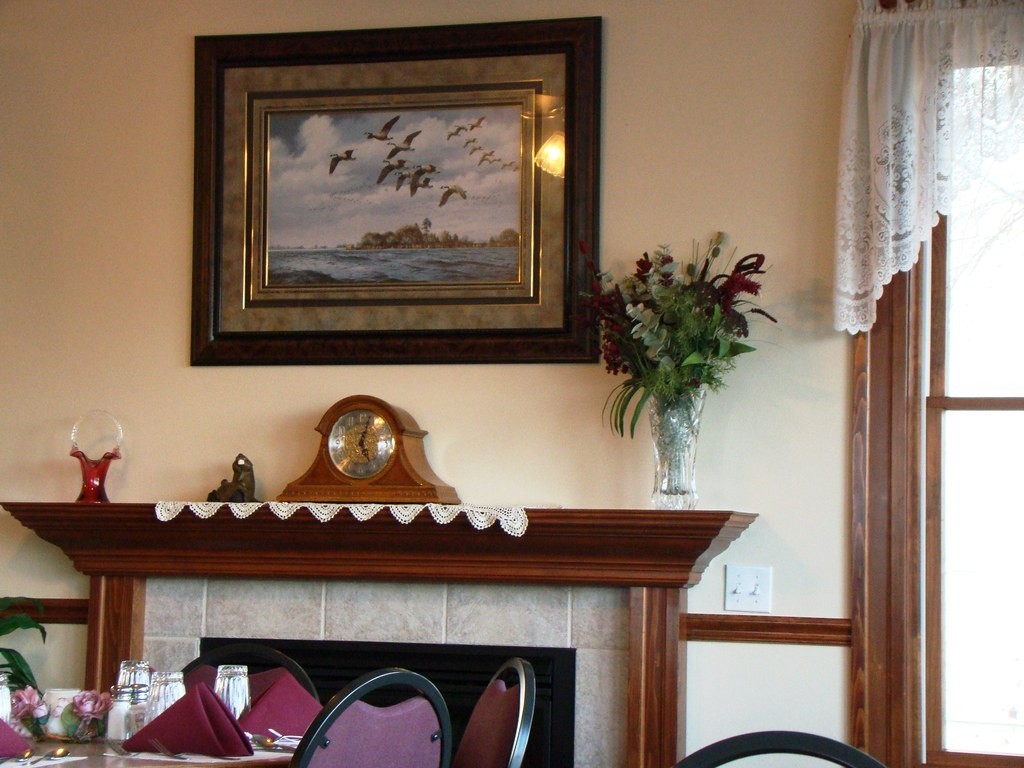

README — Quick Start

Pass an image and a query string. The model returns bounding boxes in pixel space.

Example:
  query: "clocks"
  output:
[275,393,461,505]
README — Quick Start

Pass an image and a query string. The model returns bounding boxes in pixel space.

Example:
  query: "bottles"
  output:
[106,685,149,755]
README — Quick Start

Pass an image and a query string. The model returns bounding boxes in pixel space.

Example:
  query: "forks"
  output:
[106,737,139,756]
[149,738,187,759]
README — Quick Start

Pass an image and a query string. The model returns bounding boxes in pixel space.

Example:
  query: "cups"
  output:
[0,674,10,726]
[214,664,251,721]
[148,672,185,722]
[117,660,150,686]
[39,689,82,735]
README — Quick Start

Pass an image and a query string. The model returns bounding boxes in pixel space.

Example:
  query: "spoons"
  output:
[253,734,300,747]
[22,749,70,764]
[0,749,37,760]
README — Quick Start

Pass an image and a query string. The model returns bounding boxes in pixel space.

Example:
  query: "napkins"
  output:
[235,667,325,739]
[182,664,220,694]
[119,682,256,757]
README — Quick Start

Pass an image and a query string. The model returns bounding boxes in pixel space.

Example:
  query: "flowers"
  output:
[570,226,778,475]
[1,593,114,741]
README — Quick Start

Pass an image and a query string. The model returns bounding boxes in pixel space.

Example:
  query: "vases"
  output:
[642,380,707,511]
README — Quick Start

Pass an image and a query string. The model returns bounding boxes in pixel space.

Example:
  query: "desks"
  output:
[0,744,297,768]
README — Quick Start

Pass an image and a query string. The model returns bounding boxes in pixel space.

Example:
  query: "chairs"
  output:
[184,645,893,768]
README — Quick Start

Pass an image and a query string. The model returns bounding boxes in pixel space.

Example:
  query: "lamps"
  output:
[532,129,566,180]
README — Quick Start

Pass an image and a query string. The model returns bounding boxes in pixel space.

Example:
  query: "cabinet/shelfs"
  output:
[186,14,602,367]
